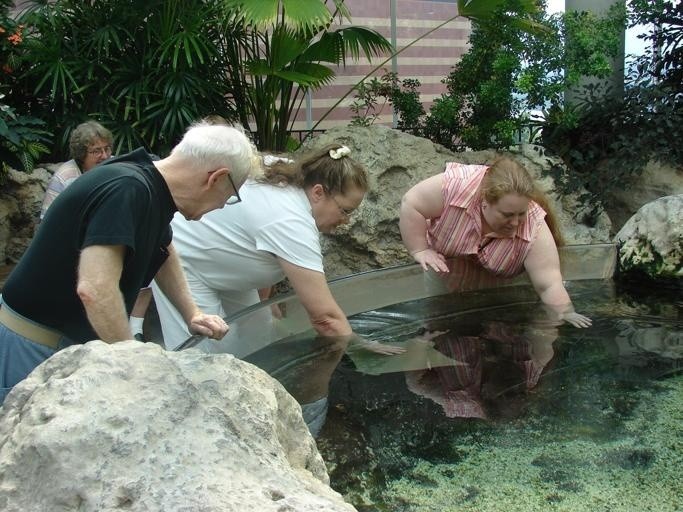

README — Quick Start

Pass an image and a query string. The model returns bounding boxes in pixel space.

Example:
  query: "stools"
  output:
[0,304,65,350]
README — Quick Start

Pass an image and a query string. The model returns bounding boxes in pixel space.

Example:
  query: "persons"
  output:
[292,337,350,439]
[36,118,152,347]
[1,122,254,407]
[397,160,608,329]
[402,294,565,427]
[130,143,406,357]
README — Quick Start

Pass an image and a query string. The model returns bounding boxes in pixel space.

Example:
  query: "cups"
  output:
[89,146,113,155]
[208,171,242,205]
